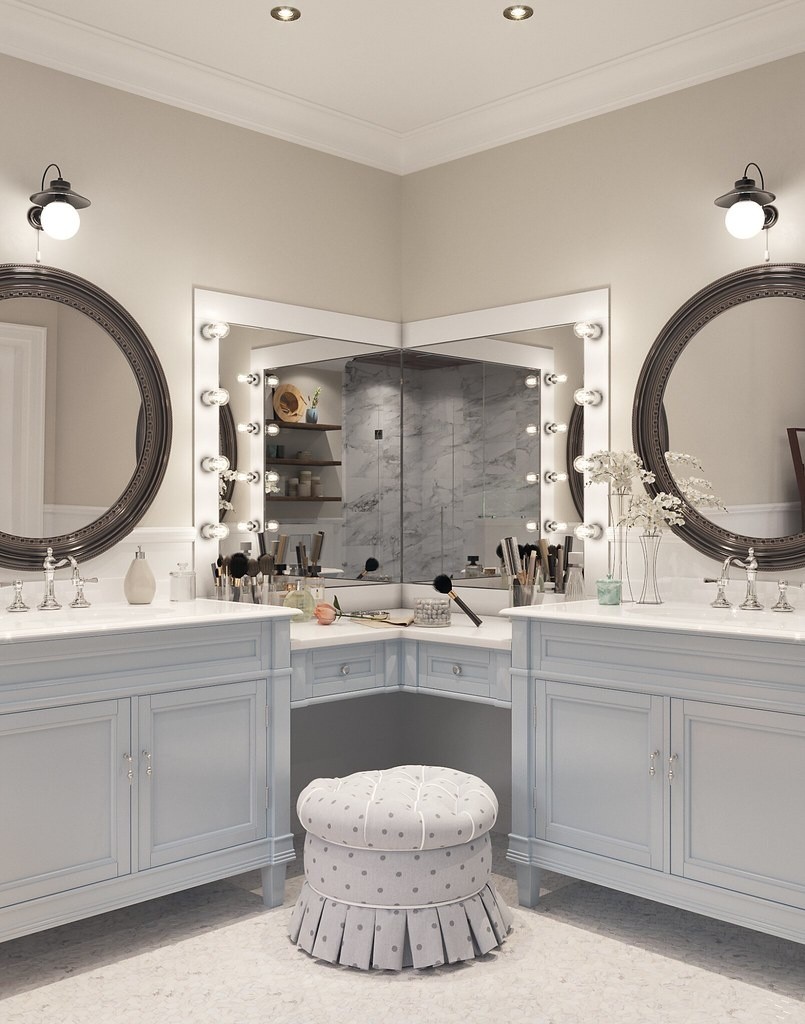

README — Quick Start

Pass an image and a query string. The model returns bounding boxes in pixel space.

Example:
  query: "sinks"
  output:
[626,605,805,629]
[0,606,175,630]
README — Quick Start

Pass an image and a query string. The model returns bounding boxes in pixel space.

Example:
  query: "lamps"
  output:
[713,162,779,239]
[28,164,94,239]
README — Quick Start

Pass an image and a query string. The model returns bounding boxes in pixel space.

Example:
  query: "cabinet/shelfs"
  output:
[505,618,805,944]
[264,419,344,502]
[0,619,294,945]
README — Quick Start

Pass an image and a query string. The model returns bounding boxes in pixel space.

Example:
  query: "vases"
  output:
[611,520,635,603]
[635,535,662,605]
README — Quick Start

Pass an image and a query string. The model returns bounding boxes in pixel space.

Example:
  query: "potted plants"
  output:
[300,388,321,423]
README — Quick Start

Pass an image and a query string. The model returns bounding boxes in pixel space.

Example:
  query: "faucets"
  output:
[720,546,766,611]
[36,546,79,611]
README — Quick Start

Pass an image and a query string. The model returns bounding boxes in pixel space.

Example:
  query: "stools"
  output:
[296,763,514,970]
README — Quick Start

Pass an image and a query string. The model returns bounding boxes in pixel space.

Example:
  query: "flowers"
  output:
[632,492,686,535]
[664,452,729,515]
[316,597,405,625]
[582,452,654,523]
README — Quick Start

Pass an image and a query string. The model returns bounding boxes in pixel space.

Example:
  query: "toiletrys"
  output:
[123,545,157,605]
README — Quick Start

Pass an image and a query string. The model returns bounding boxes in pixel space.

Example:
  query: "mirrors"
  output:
[193,288,401,599]
[402,287,611,591]
[630,263,805,572]
[0,264,173,572]
